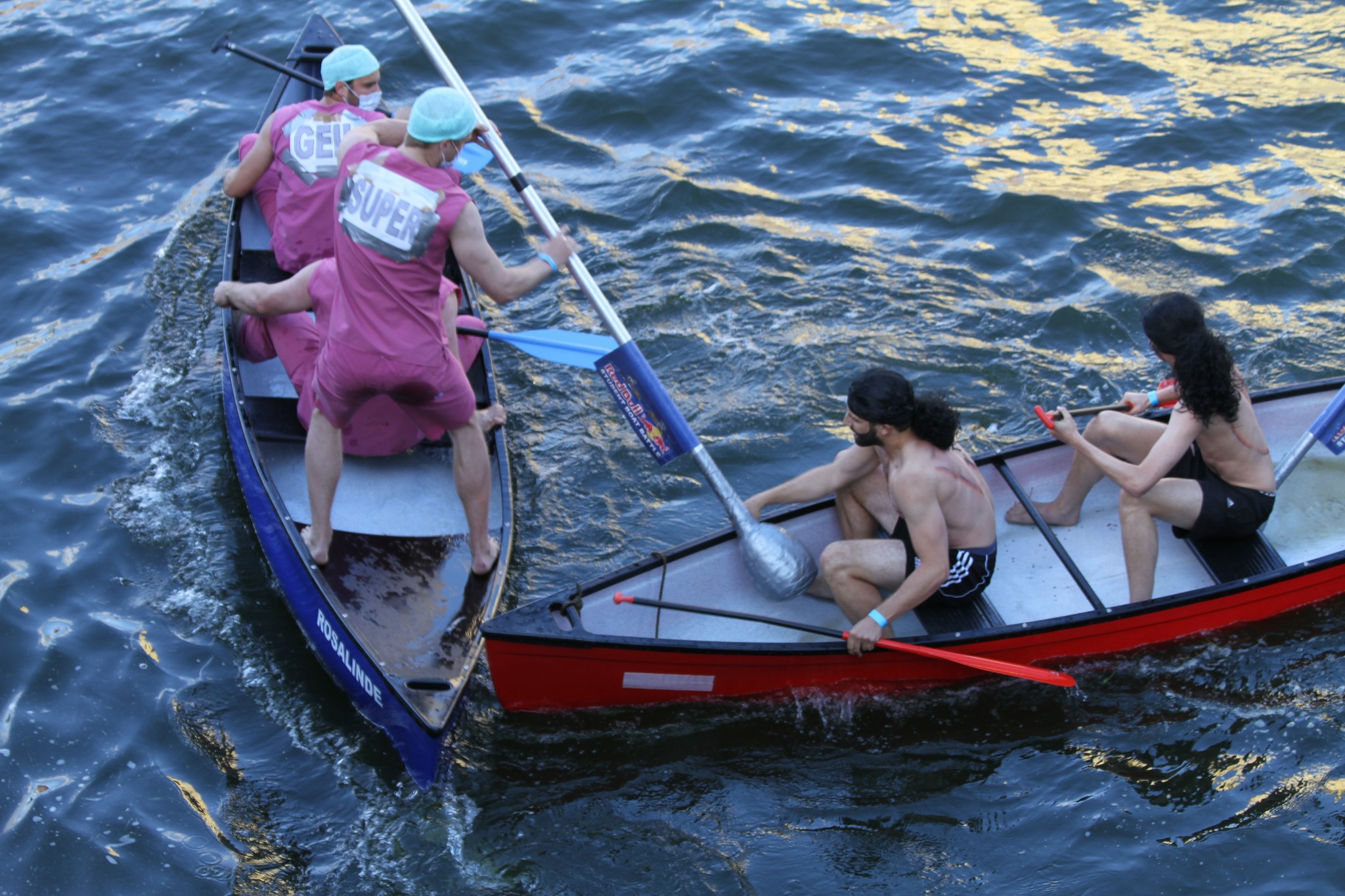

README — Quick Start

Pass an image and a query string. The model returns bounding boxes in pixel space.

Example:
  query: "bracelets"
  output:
[867,609,888,628]
[537,253,558,272]
[1147,390,1159,406]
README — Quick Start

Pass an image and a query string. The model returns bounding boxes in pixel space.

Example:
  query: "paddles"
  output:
[1036,381,1185,429]
[453,327,622,371]
[210,33,494,174]
[611,592,1076,688]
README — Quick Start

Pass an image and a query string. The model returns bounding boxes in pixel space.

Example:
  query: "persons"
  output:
[1004,291,1276,605]
[223,43,412,273]
[212,256,486,456]
[729,368,997,656]
[300,86,583,573]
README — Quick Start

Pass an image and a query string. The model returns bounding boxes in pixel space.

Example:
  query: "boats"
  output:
[217,10,515,794]
[481,374,1345,713]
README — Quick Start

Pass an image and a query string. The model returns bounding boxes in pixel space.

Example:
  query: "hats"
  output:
[406,87,478,143]
[321,44,380,91]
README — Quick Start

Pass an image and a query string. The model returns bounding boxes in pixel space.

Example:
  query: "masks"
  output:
[343,82,381,111]
[438,140,460,171]
